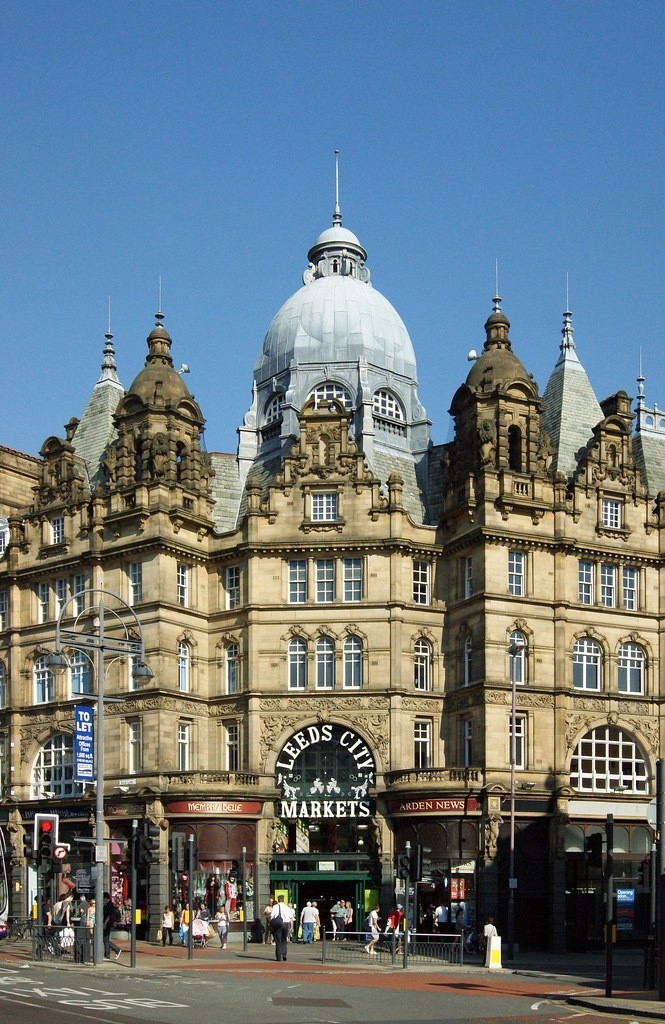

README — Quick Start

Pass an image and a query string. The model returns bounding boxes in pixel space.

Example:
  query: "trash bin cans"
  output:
[74,927,91,962]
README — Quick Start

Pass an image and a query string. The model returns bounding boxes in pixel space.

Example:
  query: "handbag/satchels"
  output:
[156,928,163,941]
[298,923,303,938]
[270,914,283,931]
[179,925,183,939]
[58,927,75,947]
[362,919,371,931]
[466,933,479,944]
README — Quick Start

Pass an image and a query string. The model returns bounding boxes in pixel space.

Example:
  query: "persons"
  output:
[424,900,449,943]
[330,900,353,942]
[225,874,239,913]
[364,903,413,954]
[451,908,473,955]
[204,874,221,920]
[160,902,230,949]
[150,437,170,477]
[179,874,194,903]
[300,901,321,944]
[101,448,116,489]
[484,917,499,967]
[263,897,296,945]
[34,892,123,964]
[270,896,296,961]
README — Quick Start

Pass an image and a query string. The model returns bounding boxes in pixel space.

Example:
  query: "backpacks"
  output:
[112,906,121,922]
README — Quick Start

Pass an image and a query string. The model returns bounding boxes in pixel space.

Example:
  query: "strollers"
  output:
[192,919,209,948]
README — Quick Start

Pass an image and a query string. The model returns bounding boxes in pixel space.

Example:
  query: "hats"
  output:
[340,900,345,903]
[396,904,403,909]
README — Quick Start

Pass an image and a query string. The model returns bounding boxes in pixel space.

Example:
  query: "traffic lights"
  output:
[23,833,33,858]
[138,835,153,865]
[34,814,58,859]
[397,855,409,878]
[144,822,160,862]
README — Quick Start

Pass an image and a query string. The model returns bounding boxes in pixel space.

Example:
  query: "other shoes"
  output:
[364,946,370,954]
[394,950,403,954]
[370,951,378,955]
[62,950,70,954]
[222,944,227,949]
[277,959,281,961]
[283,955,287,961]
[466,952,473,955]
[303,941,306,944]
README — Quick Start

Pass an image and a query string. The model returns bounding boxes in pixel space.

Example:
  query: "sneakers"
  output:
[115,950,122,959]
[103,957,110,962]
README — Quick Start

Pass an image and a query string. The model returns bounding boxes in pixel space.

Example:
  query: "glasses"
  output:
[92,903,96,904]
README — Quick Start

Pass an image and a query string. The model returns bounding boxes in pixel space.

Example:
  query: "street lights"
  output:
[41,585,154,966]
[509,641,524,942]
